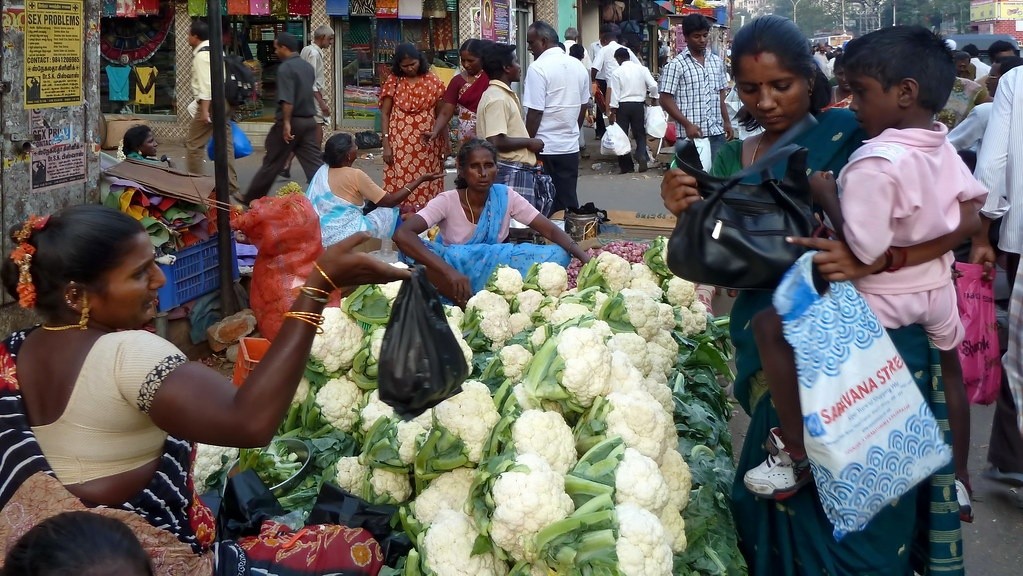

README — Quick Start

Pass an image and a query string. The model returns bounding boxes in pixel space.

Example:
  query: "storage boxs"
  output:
[155,231,240,313]
[101,118,146,149]
[233,336,271,387]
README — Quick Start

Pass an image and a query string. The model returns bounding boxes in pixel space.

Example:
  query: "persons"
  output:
[0,510,154,576]
[118,122,172,168]
[659,13,733,145]
[379,21,590,215]
[743,24,990,523]
[305,131,447,248]
[660,17,983,576]
[588,22,662,176]
[808,34,853,101]
[392,139,593,304]
[233,24,334,205]
[0,205,426,509]
[184,17,238,195]
[947,38,1023,507]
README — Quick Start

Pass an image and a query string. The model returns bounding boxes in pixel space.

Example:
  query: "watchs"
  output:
[381,133,389,138]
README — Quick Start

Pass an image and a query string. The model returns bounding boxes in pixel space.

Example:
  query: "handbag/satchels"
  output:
[600,122,632,156]
[643,105,667,138]
[592,82,606,113]
[686,136,712,173]
[600,0,668,67]
[955,261,1002,406]
[665,138,831,295]
[772,251,953,543]
[379,265,469,416]
[207,120,253,161]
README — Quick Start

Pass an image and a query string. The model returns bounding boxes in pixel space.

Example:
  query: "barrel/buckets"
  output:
[565,213,603,244]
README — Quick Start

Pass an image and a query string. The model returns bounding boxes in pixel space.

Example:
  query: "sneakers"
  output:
[956,479,975,523]
[745,427,814,500]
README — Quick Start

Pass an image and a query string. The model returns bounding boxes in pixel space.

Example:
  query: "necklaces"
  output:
[42,324,84,330]
[466,187,475,224]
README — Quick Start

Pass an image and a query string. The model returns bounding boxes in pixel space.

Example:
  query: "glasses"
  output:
[986,73,999,81]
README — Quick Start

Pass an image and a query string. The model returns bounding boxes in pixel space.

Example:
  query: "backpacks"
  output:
[198,46,255,106]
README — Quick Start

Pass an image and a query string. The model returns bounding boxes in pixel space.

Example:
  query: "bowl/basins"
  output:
[224,438,310,497]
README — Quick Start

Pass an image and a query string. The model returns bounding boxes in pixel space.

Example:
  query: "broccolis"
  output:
[190,443,241,496]
[282,235,708,576]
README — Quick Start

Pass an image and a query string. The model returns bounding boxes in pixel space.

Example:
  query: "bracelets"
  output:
[870,245,908,275]
[284,311,325,334]
[568,241,579,259]
[312,260,339,290]
[404,186,413,194]
[301,286,332,303]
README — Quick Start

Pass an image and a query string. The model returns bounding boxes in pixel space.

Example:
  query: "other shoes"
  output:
[619,165,635,174]
[986,466,1023,487]
[596,136,600,139]
[580,146,590,158]
[638,156,647,173]
[278,168,291,178]
[232,193,251,207]
